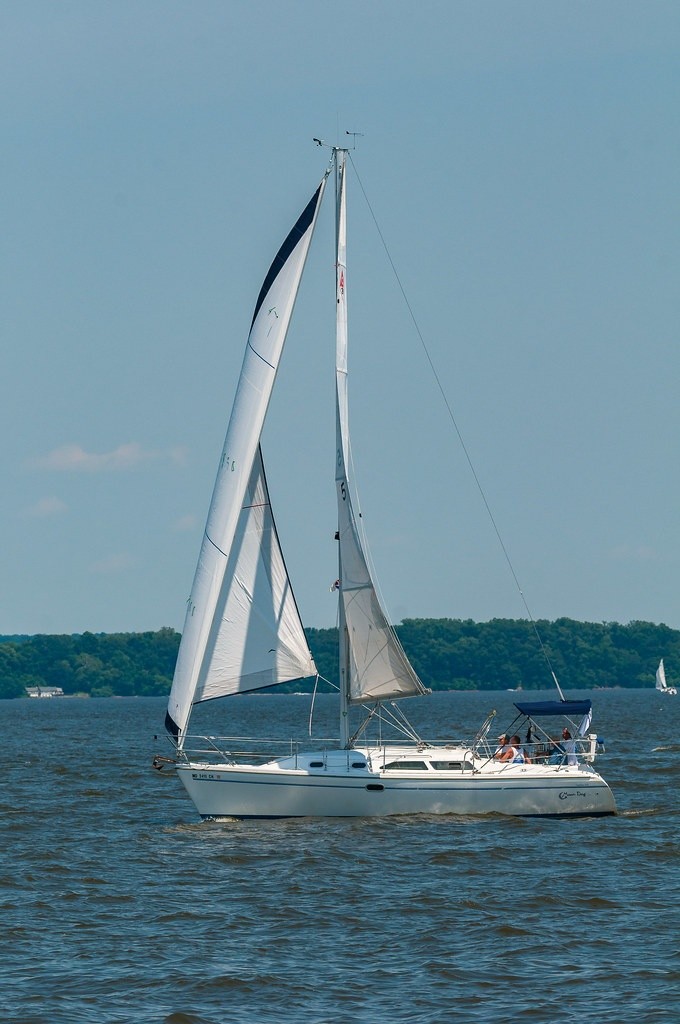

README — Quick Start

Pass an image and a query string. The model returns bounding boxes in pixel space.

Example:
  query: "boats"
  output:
[24,686,64,698]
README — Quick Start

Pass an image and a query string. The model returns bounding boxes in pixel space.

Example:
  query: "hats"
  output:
[498,734,510,741]
[563,727,574,739]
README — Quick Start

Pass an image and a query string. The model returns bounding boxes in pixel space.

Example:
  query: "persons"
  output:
[494,727,578,765]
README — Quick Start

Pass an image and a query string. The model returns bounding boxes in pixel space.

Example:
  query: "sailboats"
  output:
[655,658,677,696]
[150,128,621,819]
